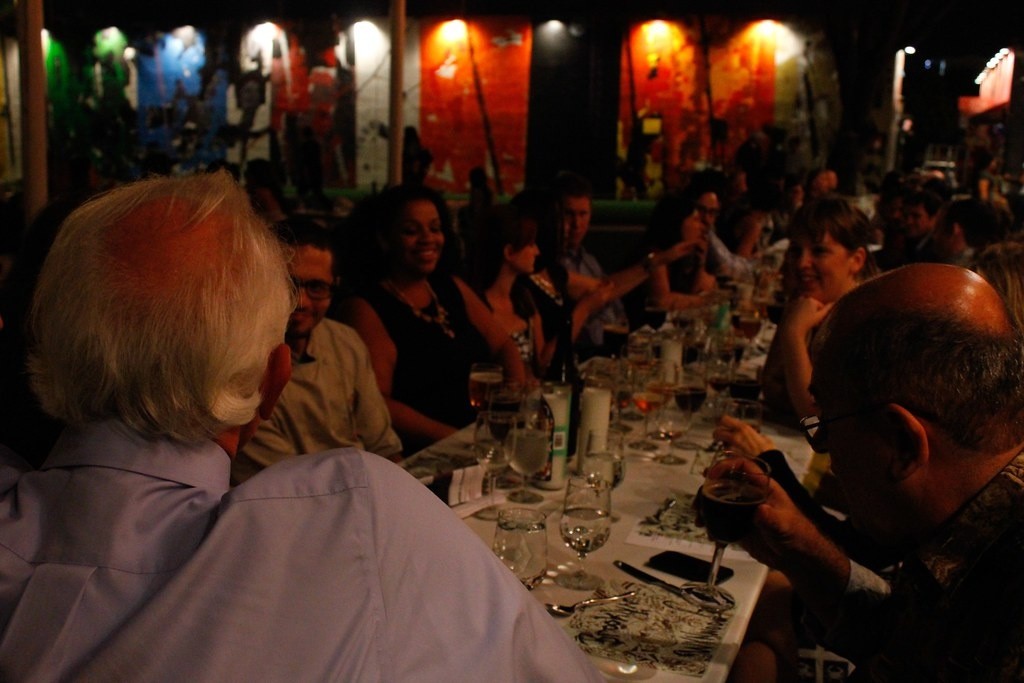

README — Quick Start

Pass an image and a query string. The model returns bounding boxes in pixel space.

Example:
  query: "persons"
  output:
[0,89,1024,682]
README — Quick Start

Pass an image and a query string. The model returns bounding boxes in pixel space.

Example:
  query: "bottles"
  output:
[540,313,583,457]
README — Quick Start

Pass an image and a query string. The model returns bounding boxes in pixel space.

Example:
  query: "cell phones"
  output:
[649,550,734,586]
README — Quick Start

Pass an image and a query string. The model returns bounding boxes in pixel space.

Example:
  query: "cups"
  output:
[709,325,767,445]
[491,508,547,591]
[580,357,623,426]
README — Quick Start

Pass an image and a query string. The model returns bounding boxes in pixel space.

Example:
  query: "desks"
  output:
[395,239,826,683]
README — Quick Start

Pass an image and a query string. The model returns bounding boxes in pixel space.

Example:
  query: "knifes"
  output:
[614,560,684,596]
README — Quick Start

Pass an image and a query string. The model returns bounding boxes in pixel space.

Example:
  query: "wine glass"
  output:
[739,306,763,356]
[681,450,771,613]
[626,360,708,465]
[627,332,653,396]
[553,475,612,590]
[671,301,695,372]
[468,362,549,522]
[584,429,626,523]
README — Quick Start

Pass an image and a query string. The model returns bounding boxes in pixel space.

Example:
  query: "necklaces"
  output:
[533,270,563,307]
[387,277,454,339]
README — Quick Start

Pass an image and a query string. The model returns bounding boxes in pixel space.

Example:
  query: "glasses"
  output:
[799,400,938,455]
[291,277,337,300]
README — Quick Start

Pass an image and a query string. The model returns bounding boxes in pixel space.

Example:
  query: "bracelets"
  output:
[640,252,658,281]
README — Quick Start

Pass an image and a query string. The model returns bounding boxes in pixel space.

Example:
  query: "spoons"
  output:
[545,591,635,615]
[645,498,675,524]
